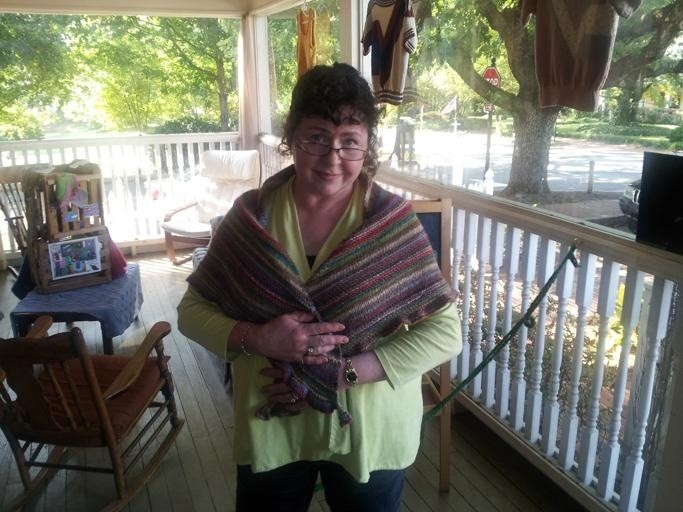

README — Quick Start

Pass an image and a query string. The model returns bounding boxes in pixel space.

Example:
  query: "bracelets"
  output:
[239,322,256,359]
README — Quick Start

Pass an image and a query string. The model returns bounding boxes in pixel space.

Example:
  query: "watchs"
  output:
[343,355,359,388]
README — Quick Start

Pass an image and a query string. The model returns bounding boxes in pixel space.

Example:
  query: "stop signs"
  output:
[482,67,502,88]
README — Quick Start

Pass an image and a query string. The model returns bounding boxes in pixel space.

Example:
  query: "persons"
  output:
[171,65,463,511]
[51,249,80,279]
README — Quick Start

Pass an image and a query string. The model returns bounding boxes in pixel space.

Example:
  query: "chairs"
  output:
[161,150,263,266]
[407,197,452,495]
[1,164,49,256]
[0,315,185,512]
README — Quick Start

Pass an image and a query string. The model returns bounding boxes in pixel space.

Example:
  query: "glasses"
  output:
[291,133,370,161]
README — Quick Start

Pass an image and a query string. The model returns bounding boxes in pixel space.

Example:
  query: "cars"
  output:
[618,176,643,234]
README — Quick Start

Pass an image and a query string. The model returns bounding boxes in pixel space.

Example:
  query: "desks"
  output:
[9,262,140,355]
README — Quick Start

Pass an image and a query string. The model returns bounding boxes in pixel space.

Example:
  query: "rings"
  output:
[290,392,299,403]
[299,356,305,366]
[306,347,314,356]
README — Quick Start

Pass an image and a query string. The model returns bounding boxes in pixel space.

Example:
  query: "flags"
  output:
[441,95,457,114]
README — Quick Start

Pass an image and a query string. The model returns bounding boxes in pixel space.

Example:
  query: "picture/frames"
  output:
[32,226,113,294]
